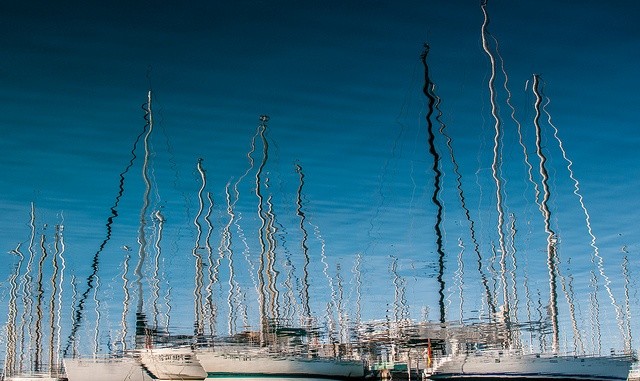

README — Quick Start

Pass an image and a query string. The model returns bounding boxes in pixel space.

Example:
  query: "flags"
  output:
[427,336,431,368]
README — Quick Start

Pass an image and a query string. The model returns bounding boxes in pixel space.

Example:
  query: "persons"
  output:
[332,341,343,361]
[381,370,393,381]
[423,373,426,381]
